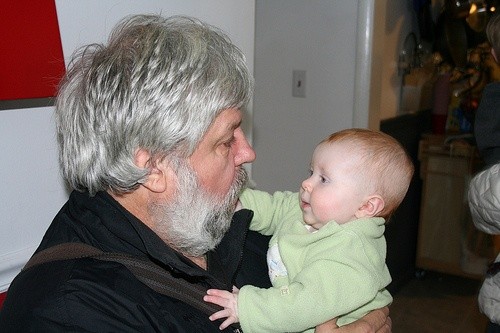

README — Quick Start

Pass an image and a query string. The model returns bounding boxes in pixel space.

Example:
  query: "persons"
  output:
[467,163,500,333]
[0,14,394,333]
[203,128,415,333]
[471,10,500,177]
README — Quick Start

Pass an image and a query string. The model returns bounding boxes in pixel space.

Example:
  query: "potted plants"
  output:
[413,0,500,281]
